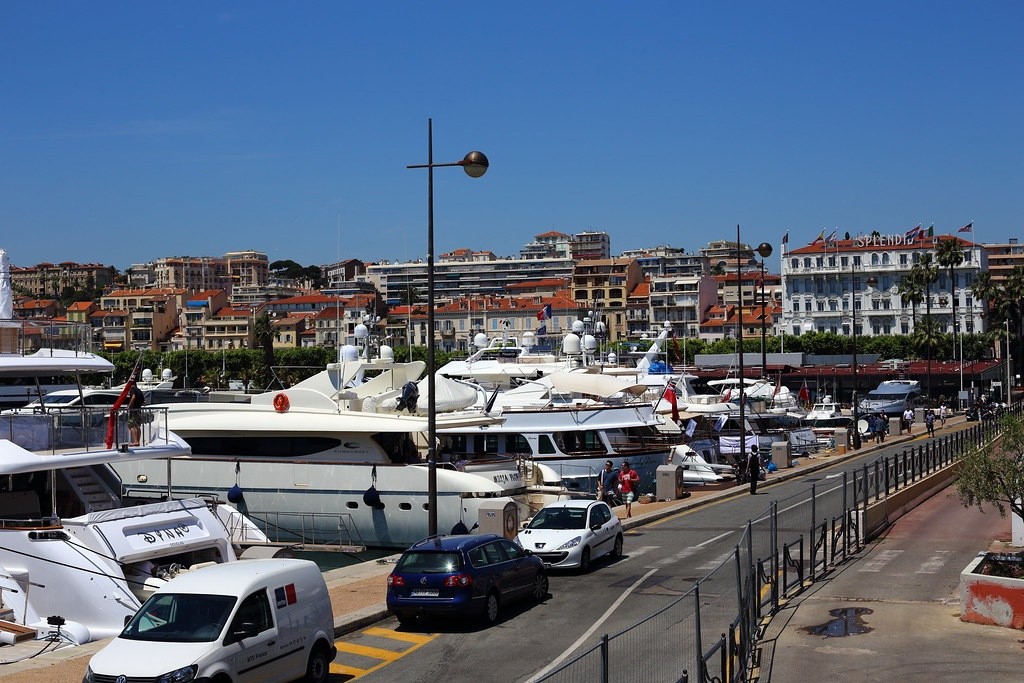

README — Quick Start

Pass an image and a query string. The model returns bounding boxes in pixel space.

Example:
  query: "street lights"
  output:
[730,225,774,483]
[407,119,490,541]
[970,298,987,405]
[922,281,949,409]
[850,262,878,449]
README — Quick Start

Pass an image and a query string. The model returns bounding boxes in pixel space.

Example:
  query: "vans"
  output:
[81,557,338,683]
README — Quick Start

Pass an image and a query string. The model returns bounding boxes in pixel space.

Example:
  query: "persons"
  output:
[125,381,144,447]
[746,444,761,495]
[868,403,947,444]
[597,460,640,519]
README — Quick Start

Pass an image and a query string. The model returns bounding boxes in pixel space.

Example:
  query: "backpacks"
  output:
[749,452,759,469]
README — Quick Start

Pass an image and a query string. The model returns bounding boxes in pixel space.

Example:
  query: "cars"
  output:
[512,499,623,570]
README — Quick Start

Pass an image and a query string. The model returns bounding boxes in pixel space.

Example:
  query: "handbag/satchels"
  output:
[595,486,603,501]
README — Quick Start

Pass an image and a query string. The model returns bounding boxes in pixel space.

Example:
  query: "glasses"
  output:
[606,464,610,466]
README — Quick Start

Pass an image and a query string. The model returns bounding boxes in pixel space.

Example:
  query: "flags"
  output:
[536,306,552,320]
[772,375,781,397]
[799,378,810,401]
[905,221,972,240]
[106,362,142,450]
[782,231,839,246]
[720,389,732,403]
[662,381,680,424]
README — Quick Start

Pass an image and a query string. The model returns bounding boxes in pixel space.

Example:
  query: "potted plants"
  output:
[951,401,1024,629]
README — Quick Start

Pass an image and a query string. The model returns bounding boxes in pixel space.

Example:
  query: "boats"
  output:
[617,369,918,485]
[0,319,236,663]
[132,315,669,546]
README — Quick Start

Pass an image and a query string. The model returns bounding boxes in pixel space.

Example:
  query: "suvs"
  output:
[386,534,549,622]
